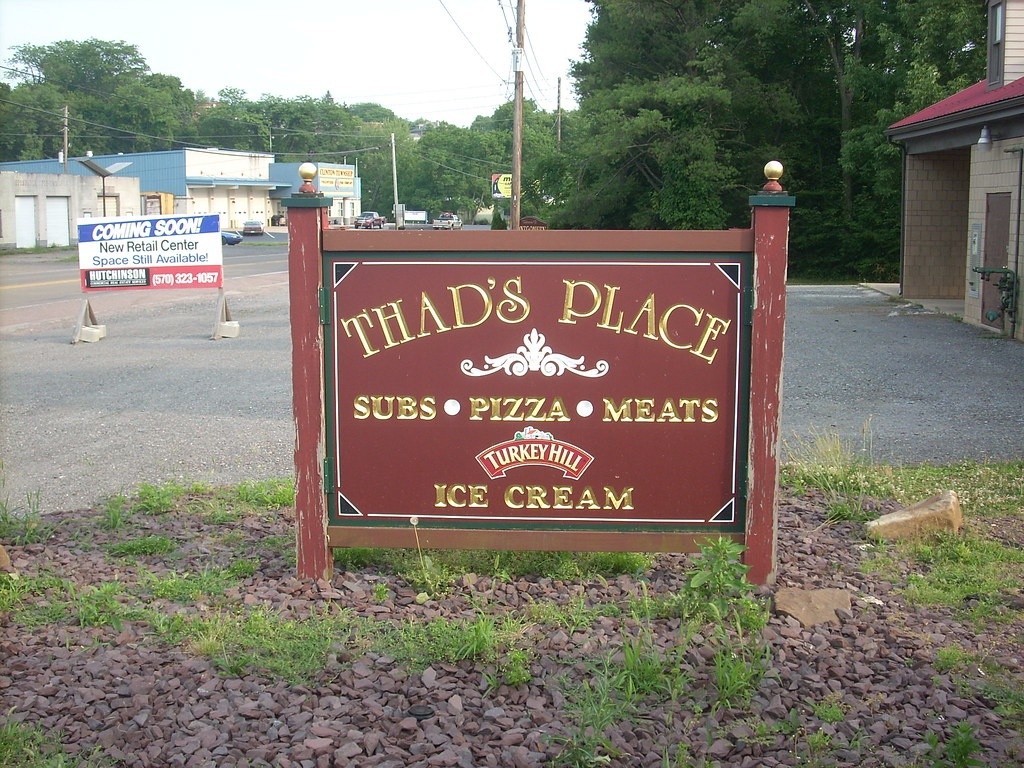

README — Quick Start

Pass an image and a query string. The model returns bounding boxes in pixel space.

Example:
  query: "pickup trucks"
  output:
[354,212,386,229]
[433,213,462,231]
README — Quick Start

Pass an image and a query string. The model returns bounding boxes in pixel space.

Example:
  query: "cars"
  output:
[243,221,264,236]
[222,232,244,245]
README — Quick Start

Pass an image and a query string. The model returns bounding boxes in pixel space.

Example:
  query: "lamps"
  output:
[977,124,1000,151]
